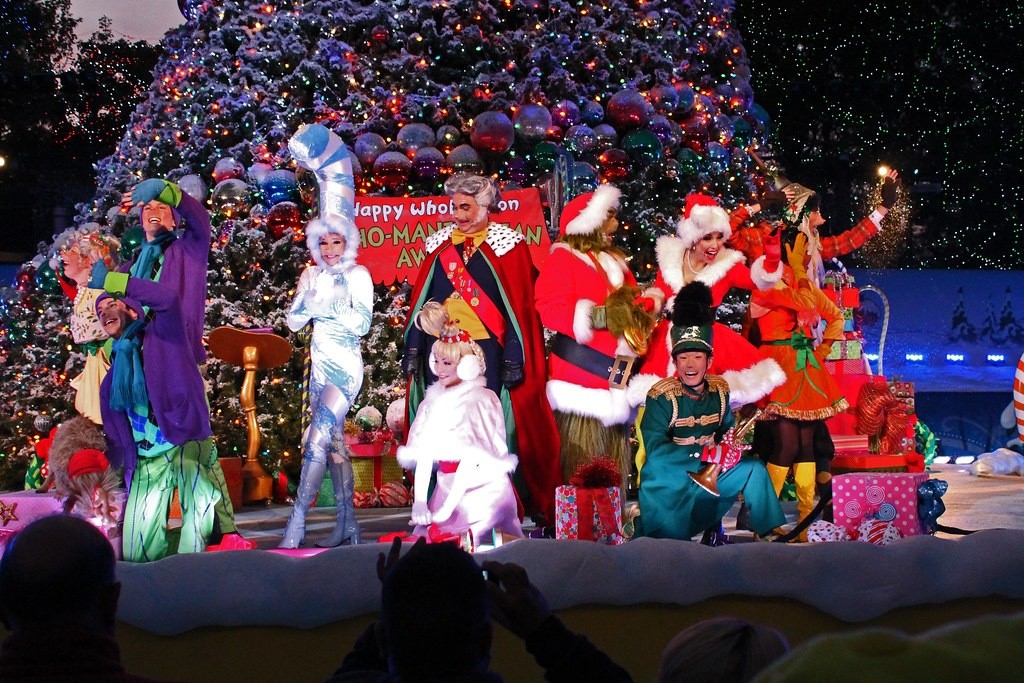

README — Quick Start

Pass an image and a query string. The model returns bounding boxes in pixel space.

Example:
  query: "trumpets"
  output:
[622,303,658,356]
[684,408,766,497]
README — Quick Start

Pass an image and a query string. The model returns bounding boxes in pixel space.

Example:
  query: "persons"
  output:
[62,178,238,562]
[395,299,525,550]
[325,537,632,683]
[657,618,788,683]
[0,514,166,683]
[279,232,373,548]
[404,176,899,547]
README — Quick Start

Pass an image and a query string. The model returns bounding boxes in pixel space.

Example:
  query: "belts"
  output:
[545,328,641,384]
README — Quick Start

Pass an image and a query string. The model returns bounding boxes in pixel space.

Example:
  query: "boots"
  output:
[765,460,789,535]
[277,460,326,549]
[315,459,362,549]
[793,461,816,541]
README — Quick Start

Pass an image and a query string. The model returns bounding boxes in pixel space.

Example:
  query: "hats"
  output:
[287,123,360,265]
[557,183,619,237]
[95,291,144,321]
[140,200,180,233]
[679,193,732,245]
[670,280,713,354]
[767,169,815,223]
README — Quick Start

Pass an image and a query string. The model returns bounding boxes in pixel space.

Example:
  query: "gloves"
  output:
[633,296,655,314]
[401,349,420,382]
[410,504,432,527]
[762,227,782,273]
[434,505,452,523]
[502,360,524,390]
[815,343,832,360]
[709,443,741,469]
[784,234,810,281]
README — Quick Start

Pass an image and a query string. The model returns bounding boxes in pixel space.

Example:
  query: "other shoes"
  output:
[702,533,733,549]
[755,530,786,542]
